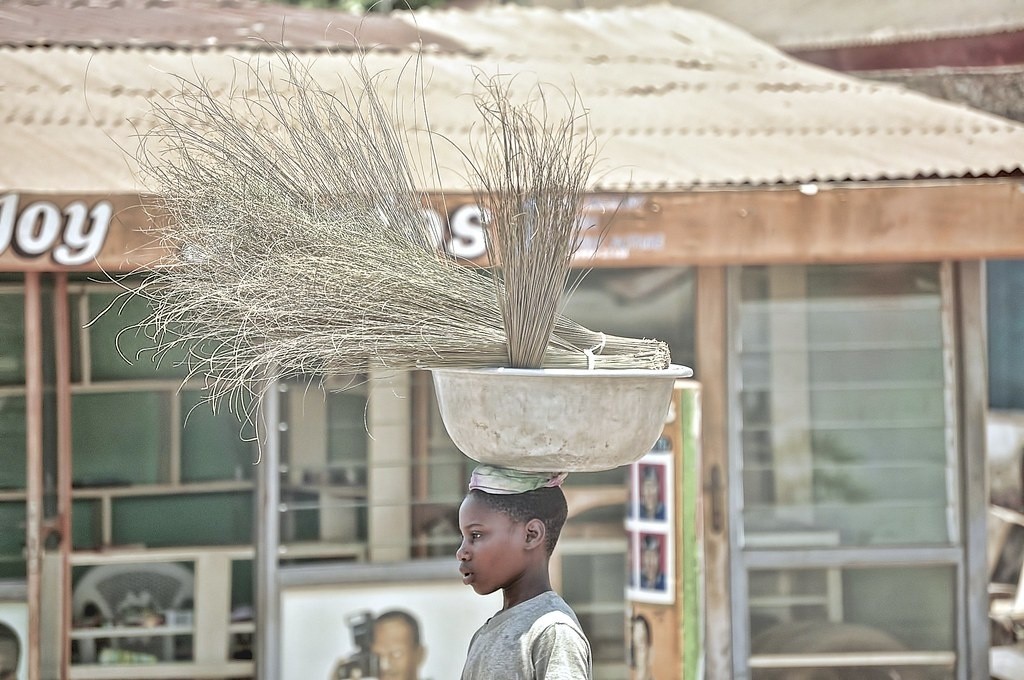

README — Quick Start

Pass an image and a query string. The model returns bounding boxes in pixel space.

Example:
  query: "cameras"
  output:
[338,612,381,680]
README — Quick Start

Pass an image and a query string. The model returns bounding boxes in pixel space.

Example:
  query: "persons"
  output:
[641,470,665,519]
[632,617,651,675]
[640,539,665,590]
[331,612,426,680]
[456,484,590,680]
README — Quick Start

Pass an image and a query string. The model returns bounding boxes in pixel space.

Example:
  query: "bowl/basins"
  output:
[422,362,694,474]
